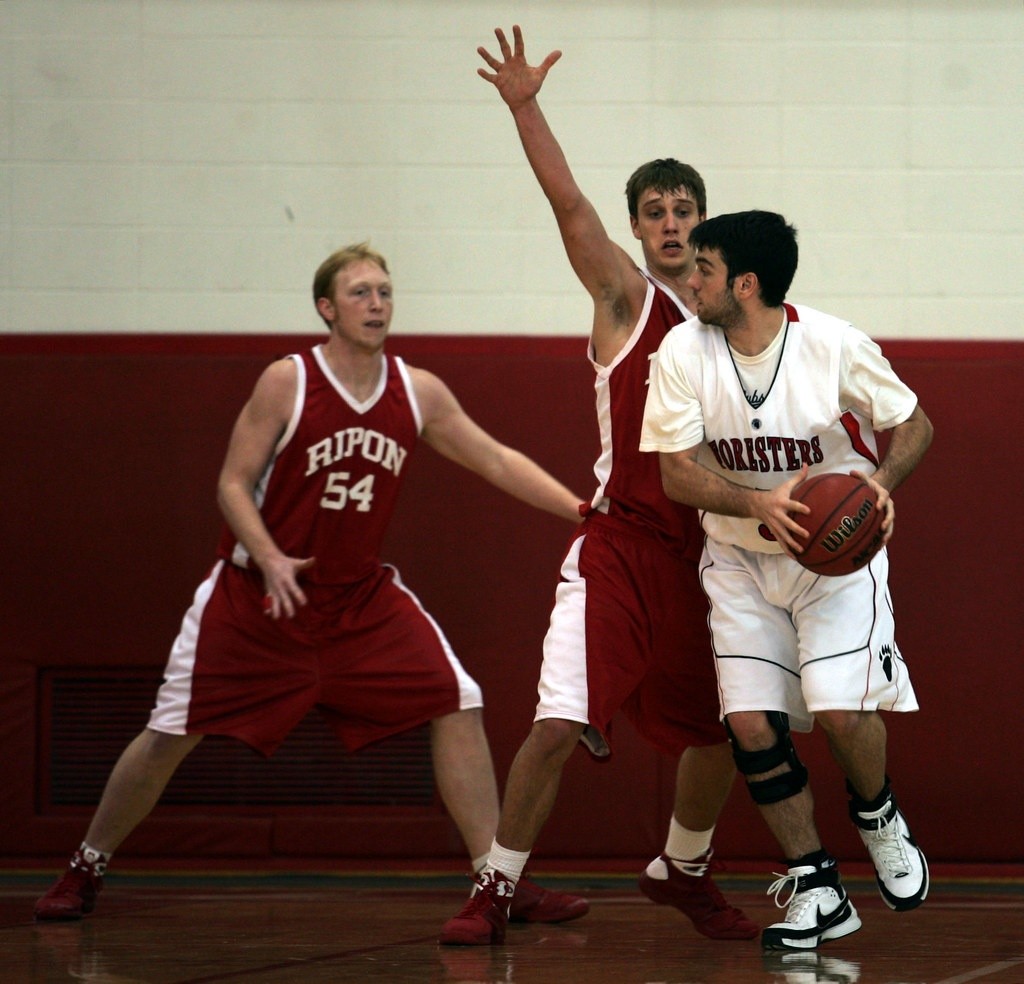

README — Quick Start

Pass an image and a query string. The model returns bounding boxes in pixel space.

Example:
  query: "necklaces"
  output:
[724,321,790,431]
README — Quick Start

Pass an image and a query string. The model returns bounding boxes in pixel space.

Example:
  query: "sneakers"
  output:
[638,845,760,941]
[436,865,517,947]
[847,783,930,911]
[507,873,590,923]
[761,848,864,952]
[34,849,106,923]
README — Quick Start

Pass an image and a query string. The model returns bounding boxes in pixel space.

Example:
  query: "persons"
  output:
[433,22,764,947]
[636,207,933,958]
[28,238,593,922]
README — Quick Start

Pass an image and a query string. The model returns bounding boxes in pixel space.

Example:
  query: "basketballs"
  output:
[778,472,885,577]
